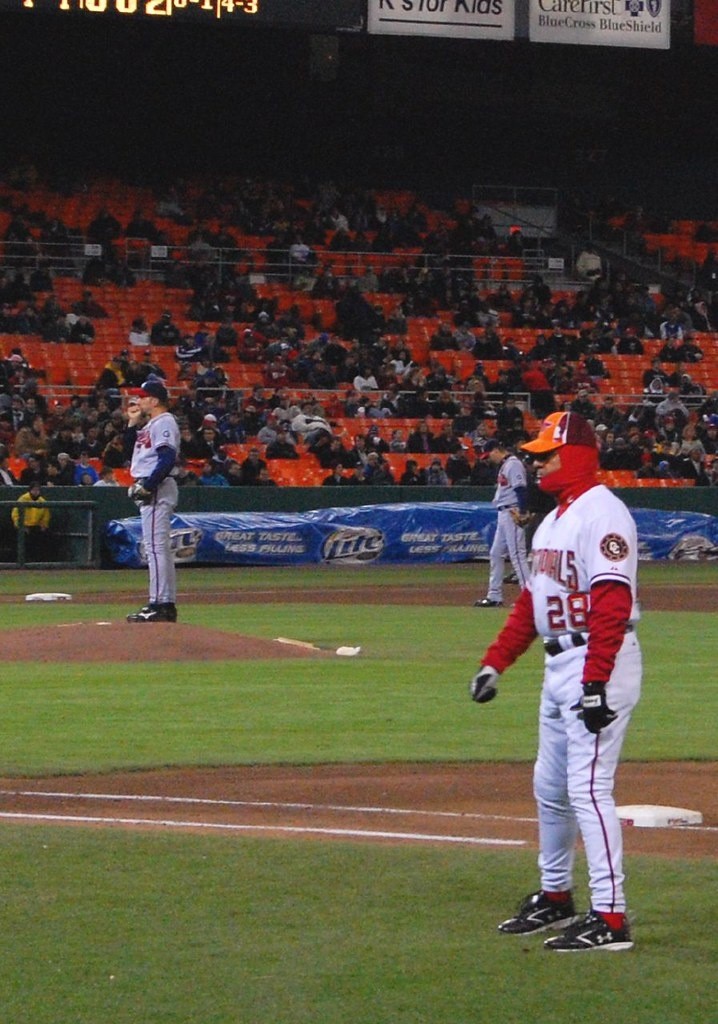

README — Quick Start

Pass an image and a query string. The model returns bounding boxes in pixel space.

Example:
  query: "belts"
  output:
[498,503,519,510]
[544,624,633,657]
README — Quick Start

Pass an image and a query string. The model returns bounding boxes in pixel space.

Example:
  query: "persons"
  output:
[121,379,181,623]
[470,411,641,953]
[474,441,530,607]
[0,178,718,562]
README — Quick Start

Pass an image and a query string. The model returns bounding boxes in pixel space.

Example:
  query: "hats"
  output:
[480,440,498,460]
[267,412,280,420]
[520,412,596,453]
[276,427,288,434]
[128,381,167,399]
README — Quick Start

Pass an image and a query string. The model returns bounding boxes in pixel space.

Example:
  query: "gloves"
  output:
[570,687,615,736]
[471,666,500,703]
[128,481,153,506]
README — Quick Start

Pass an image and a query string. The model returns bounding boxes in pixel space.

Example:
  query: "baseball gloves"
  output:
[127,480,154,509]
[510,508,533,528]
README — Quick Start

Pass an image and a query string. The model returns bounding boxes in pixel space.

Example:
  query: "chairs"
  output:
[0,168,718,488]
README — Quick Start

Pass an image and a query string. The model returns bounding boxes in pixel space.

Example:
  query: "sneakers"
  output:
[543,914,634,952]
[503,573,521,584]
[474,598,503,607]
[127,603,177,622]
[498,892,581,934]
